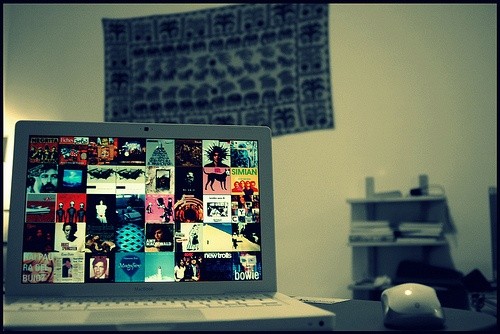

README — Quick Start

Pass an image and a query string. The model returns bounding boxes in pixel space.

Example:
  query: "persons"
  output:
[21,135,262,285]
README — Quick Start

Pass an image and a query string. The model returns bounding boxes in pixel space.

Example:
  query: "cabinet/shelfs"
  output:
[345,174,453,247]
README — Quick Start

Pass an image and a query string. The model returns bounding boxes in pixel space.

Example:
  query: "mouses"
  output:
[381,283,444,327]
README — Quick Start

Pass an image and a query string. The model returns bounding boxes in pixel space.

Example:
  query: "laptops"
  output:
[3,121,336,331]
